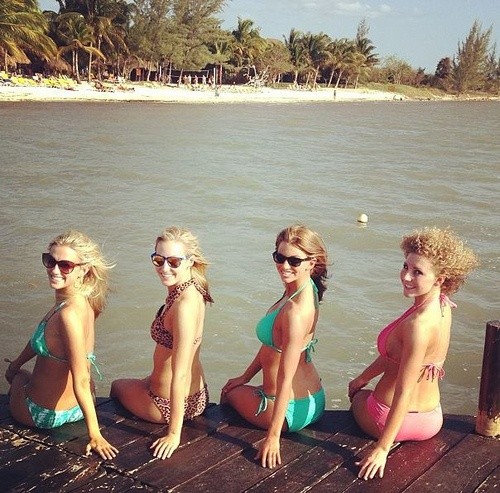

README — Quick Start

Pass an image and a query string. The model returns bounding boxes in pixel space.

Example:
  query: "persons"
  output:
[4,228,120,460]
[183,74,215,86]
[109,224,217,462]
[220,224,336,469]
[348,224,479,481]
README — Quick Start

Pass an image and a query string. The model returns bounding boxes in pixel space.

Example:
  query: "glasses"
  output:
[151,253,186,268]
[272,250,314,267]
[42,253,89,274]
